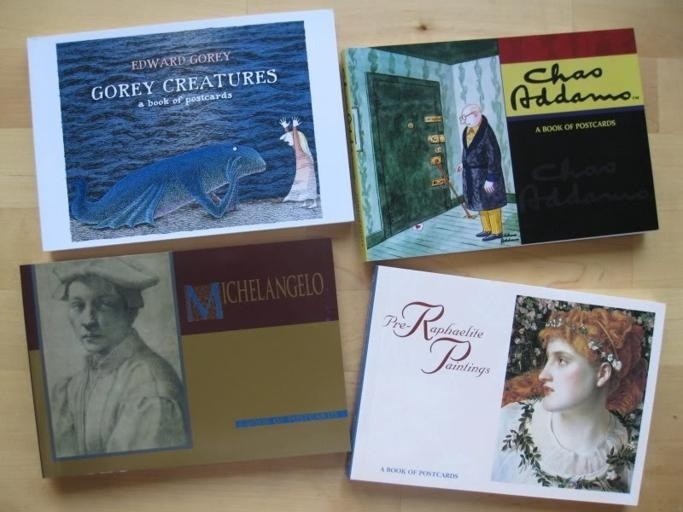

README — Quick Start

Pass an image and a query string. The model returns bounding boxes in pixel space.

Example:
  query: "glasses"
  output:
[459,112,473,119]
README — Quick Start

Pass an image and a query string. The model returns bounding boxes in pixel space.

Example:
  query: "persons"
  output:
[490,307,648,494]
[48,255,190,457]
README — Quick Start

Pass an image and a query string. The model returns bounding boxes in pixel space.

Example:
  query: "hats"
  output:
[52,258,158,308]
